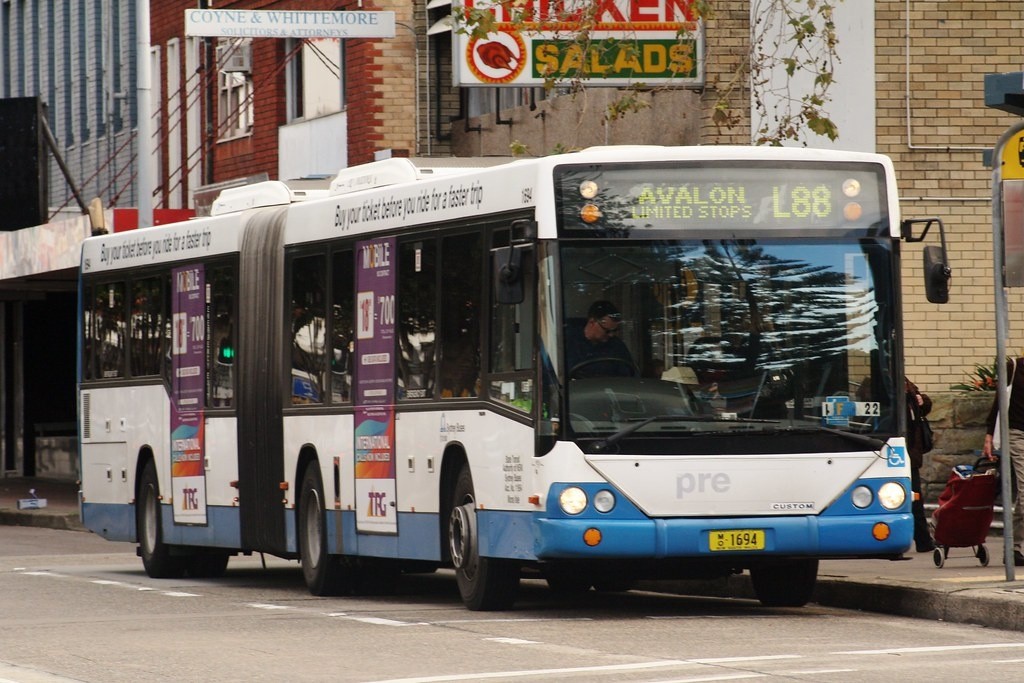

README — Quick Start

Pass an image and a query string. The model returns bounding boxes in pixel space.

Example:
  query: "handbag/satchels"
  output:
[993,356,1017,449]
[909,379,933,453]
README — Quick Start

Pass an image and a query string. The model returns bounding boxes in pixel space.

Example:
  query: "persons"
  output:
[981,356,1024,567]
[904,376,937,555]
[565,300,635,381]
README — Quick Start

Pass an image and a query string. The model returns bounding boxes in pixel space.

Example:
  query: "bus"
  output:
[72,144,954,614]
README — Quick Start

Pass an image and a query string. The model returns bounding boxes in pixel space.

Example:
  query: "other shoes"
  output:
[1005,552,1024,565]
[917,538,939,553]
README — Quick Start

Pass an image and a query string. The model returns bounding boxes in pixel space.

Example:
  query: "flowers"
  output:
[950,358,997,390]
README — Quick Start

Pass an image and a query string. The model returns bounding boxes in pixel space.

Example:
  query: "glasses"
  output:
[598,321,617,333]
